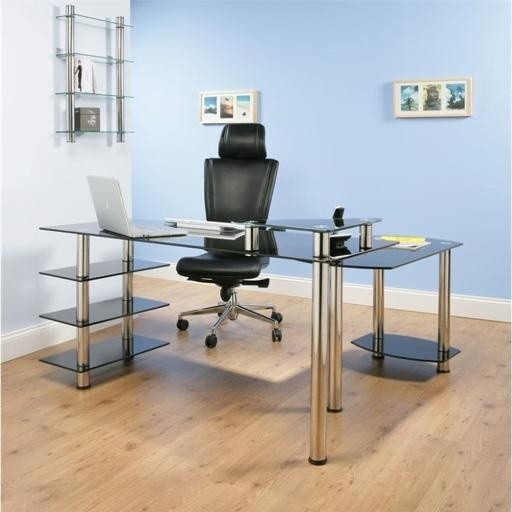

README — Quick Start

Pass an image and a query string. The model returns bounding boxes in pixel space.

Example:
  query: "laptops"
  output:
[86,175,188,239]
